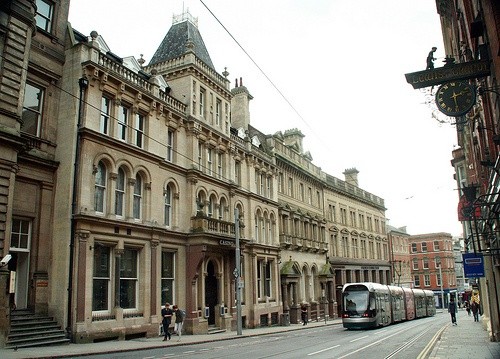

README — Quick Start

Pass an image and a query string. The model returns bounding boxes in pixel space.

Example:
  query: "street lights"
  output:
[234,208,245,335]
[439,261,444,309]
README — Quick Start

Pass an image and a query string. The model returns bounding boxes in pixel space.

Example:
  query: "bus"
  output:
[341,259,436,329]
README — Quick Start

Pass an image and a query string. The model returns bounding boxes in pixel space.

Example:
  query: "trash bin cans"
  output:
[281,313,289,326]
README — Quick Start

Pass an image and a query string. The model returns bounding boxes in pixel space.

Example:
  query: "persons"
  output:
[161,303,173,341]
[471,300,481,322]
[466,301,471,314]
[448,299,458,326]
[299,303,307,326]
[171,305,184,342]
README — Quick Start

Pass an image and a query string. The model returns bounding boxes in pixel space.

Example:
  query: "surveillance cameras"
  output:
[0,254,12,267]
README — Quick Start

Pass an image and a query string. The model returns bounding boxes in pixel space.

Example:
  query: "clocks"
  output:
[435,81,476,117]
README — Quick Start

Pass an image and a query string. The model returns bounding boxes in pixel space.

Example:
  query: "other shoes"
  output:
[163,338,167,341]
[454,322,457,325]
[453,323,454,326]
[169,334,172,340]
